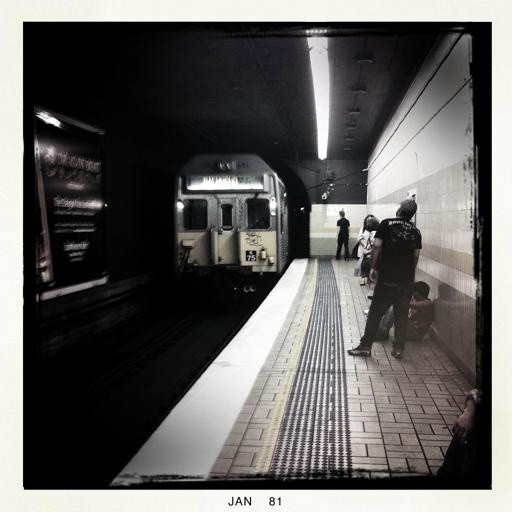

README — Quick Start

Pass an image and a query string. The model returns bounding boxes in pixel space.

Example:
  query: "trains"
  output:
[173,152,290,296]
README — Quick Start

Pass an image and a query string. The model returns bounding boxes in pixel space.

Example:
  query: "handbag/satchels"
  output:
[361,258,370,276]
[351,242,359,258]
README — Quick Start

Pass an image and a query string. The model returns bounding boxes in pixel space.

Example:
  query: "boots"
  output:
[360,278,365,285]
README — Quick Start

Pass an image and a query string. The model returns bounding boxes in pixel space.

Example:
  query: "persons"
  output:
[367,219,380,299]
[373,281,434,341]
[336,211,350,261]
[356,214,374,285]
[347,200,421,358]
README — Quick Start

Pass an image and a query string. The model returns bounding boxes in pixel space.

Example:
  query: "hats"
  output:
[401,200,417,212]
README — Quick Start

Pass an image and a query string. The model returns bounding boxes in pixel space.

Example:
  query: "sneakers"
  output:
[392,349,403,358]
[348,346,371,357]
